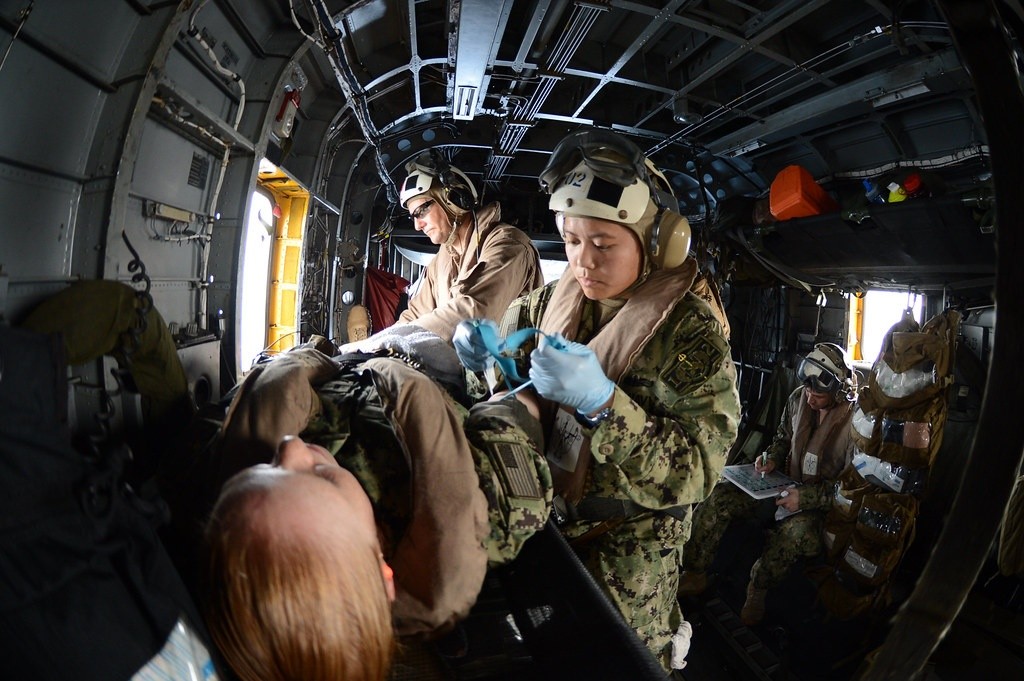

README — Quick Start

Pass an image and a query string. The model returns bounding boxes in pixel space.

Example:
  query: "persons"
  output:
[188,129,742,681]
[682,341,867,641]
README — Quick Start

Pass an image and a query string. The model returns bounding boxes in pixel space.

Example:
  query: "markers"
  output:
[760,451,768,480]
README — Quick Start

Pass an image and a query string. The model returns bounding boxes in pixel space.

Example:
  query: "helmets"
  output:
[548,132,680,225]
[399,163,479,211]
[797,343,852,391]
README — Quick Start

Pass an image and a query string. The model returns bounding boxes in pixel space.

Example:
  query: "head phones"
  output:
[815,343,859,402]
[538,129,691,270]
[405,152,476,214]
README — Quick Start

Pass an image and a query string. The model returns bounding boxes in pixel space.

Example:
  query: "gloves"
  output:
[529,333,614,414]
[452,318,503,373]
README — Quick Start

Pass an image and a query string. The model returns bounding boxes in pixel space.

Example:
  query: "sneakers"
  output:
[676,570,707,597]
[740,580,769,625]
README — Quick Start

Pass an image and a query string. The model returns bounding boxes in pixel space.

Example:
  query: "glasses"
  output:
[407,199,434,222]
[543,129,647,187]
[405,148,455,188]
[793,354,846,393]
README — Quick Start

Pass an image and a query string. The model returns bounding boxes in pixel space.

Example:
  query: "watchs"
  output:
[583,407,610,426]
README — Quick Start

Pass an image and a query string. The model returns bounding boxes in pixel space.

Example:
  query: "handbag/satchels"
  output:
[365,265,411,336]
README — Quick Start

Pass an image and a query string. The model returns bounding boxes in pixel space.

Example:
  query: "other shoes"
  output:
[346,304,373,343]
